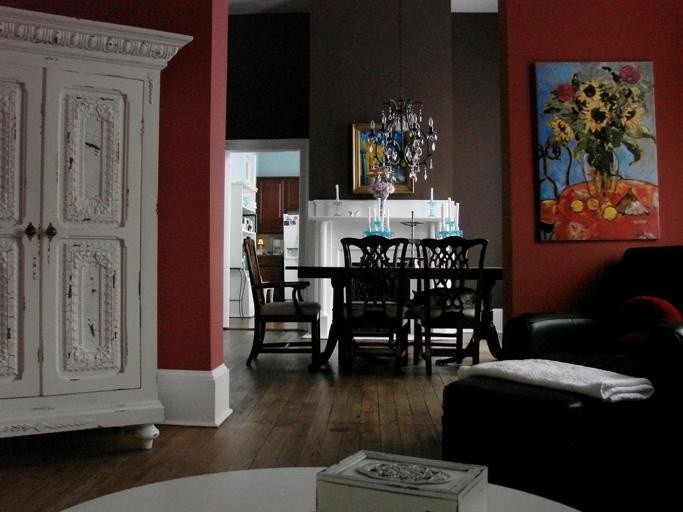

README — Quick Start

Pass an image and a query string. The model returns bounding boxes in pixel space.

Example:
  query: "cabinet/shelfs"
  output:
[231,180,258,318]
[0,6,194,451]
[256,176,300,234]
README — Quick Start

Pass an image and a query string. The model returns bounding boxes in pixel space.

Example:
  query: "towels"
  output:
[457,358,656,404]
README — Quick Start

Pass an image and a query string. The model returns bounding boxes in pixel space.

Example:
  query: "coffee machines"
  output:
[272,239,283,255]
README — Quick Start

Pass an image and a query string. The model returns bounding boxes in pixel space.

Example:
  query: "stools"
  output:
[442,375,660,512]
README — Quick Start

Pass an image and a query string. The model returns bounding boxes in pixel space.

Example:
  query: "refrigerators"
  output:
[282,213,299,300]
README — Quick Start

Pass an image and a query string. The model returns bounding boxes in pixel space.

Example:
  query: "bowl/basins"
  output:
[243,195,256,210]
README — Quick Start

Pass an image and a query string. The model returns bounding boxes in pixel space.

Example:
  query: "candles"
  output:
[335,185,459,234]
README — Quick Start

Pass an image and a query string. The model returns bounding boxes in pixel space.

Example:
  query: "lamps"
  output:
[367,2,437,182]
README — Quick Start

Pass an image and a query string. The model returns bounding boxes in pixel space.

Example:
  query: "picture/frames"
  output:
[352,122,414,195]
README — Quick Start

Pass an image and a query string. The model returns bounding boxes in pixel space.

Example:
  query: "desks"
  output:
[62,467,580,512]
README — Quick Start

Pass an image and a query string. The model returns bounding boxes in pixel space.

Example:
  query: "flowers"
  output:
[544,62,655,222]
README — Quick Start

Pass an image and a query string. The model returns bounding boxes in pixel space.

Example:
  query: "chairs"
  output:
[243,235,490,379]
[503,244,683,405]
